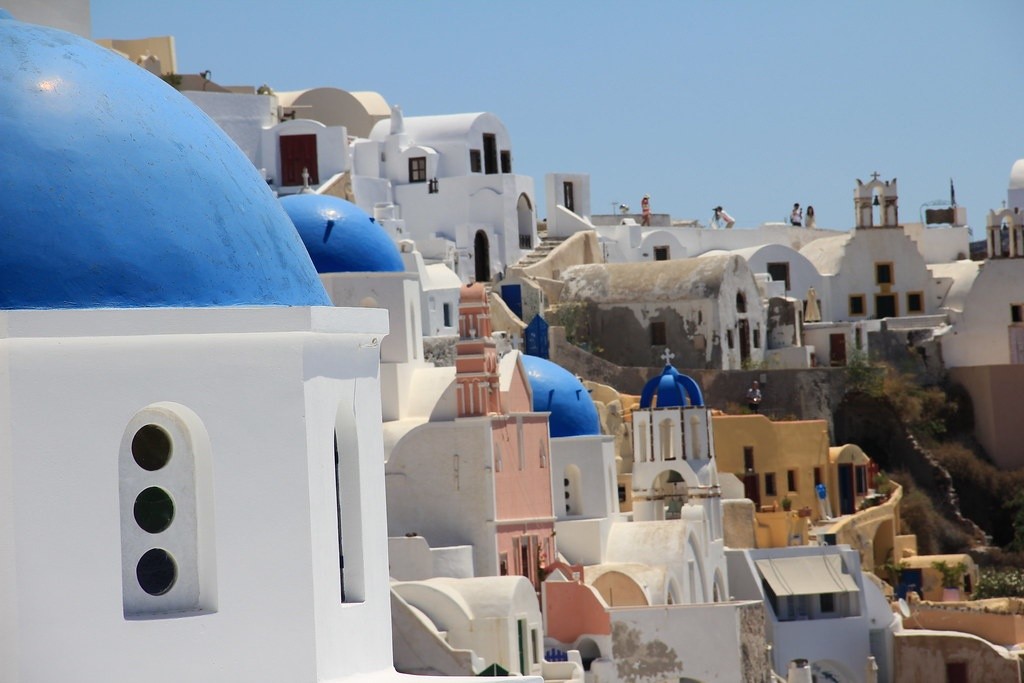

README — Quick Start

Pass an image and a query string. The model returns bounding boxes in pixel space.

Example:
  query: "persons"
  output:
[746,381,762,413]
[715,206,735,228]
[782,493,791,510]
[641,198,650,226]
[905,584,924,629]
[785,659,818,683]
[790,203,802,226]
[805,206,816,230]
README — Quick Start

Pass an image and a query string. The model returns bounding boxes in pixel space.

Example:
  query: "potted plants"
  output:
[930,559,969,601]
[782,495,792,511]
[857,469,893,511]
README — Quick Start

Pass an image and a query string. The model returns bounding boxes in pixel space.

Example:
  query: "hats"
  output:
[643,193,650,199]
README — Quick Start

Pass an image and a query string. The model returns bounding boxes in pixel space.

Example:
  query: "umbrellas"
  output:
[804,285,821,323]
